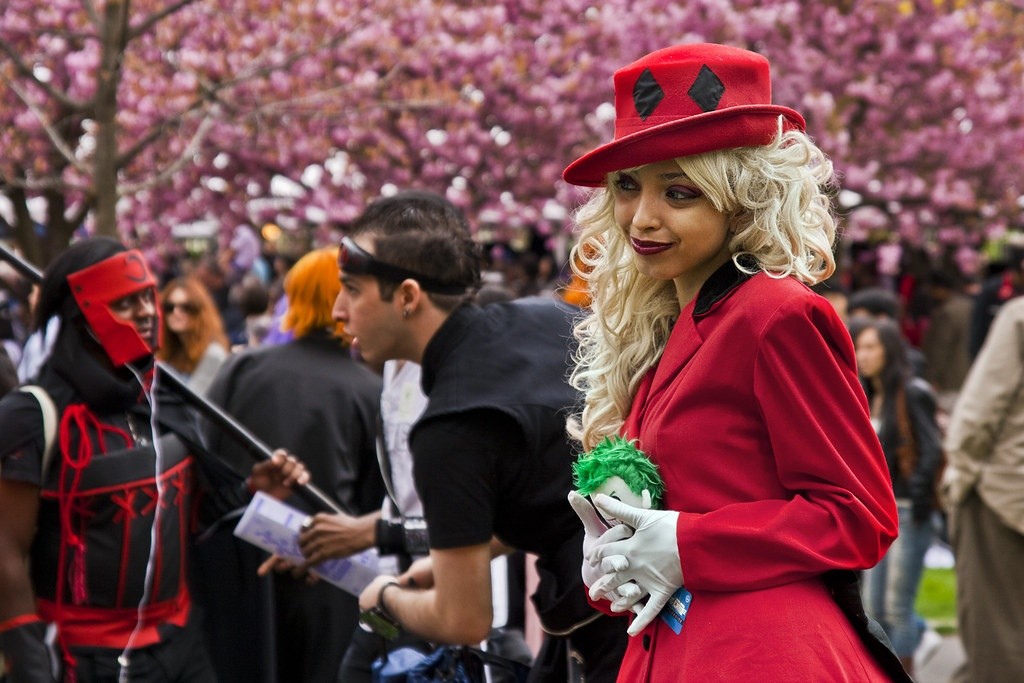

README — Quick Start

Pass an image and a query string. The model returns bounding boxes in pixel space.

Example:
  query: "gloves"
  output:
[585,493,683,637]
[567,489,645,616]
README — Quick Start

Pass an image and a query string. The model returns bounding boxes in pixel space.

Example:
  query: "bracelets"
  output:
[377,583,405,626]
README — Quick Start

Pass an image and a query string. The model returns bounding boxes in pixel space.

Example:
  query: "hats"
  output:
[562,42,805,187]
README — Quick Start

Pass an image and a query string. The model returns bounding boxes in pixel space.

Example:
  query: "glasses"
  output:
[336,236,483,297]
[161,301,198,316]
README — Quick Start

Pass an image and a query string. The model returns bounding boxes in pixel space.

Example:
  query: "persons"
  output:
[0,196,1024,683]
[561,41,899,682]
